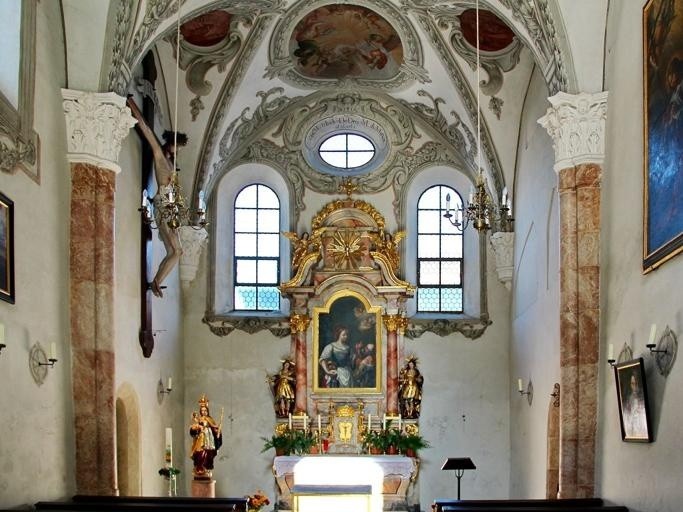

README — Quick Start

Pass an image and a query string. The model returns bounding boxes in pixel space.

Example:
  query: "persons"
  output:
[380,232,398,269]
[124,93,187,298]
[397,359,424,420]
[275,360,297,418]
[191,412,199,426]
[620,370,647,438]
[291,231,314,268]
[197,405,219,473]
[317,325,372,388]
[353,343,374,388]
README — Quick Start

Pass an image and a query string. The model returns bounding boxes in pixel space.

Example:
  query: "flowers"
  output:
[243,489,270,512]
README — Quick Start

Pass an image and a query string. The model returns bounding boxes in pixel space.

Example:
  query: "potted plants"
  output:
[360,419,434,457]
[258,434,290,456]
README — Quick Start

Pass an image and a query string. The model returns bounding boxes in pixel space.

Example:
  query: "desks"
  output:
[271,452,420,512]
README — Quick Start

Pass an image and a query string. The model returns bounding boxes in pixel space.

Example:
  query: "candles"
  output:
[164,426,174,465]
[608,342,614,360]
[648,323,656,344]
[168,377,172,388]
[288,412,402,433]
[518,378,522,390]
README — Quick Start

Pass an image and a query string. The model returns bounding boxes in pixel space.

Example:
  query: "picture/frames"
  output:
[0,190,17,307]
[0,0,37,176]
[613,356,654,444]
[639,0,683,277]
[311,289,382,394]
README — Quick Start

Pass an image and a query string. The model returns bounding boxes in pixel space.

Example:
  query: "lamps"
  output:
[443,0,516,236]
[440,457,476,501]
[137,0,211,234]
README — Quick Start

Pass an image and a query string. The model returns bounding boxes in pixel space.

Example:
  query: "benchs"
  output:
[34,494,250,512]
[290,483,373,512]
[431,496,630,512]
